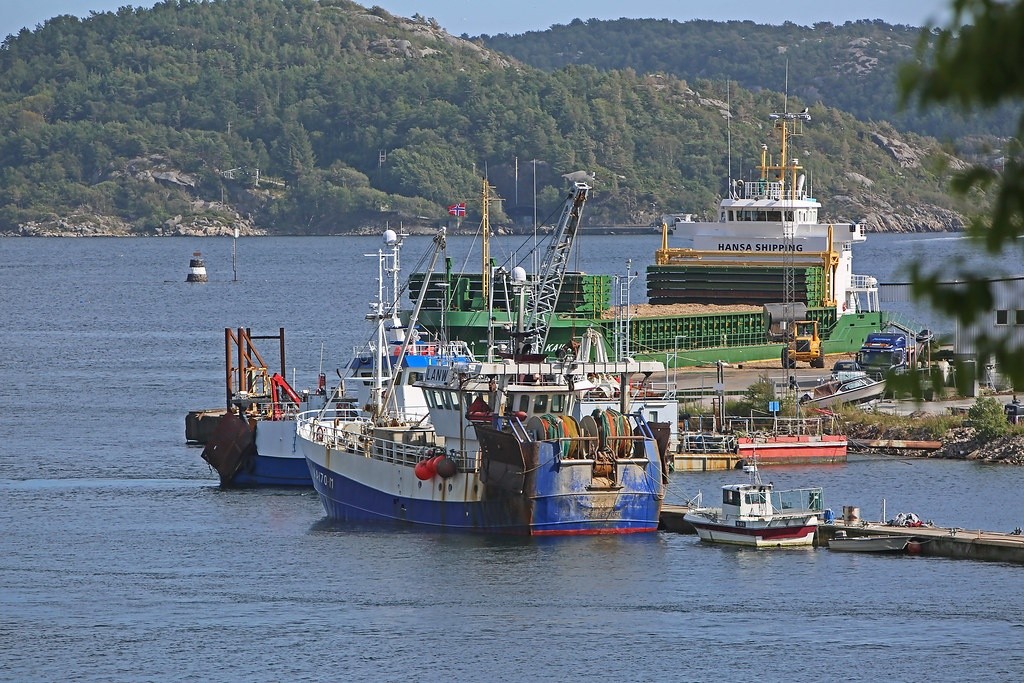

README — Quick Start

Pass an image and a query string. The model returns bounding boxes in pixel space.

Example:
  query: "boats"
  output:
[205,156,678,535]
[827,535,915,551]
[799,373,886,409]
[398,59,889,364]
[682,444,823,548]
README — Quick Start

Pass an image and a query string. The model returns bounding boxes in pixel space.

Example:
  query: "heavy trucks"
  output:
[856,333,907,382]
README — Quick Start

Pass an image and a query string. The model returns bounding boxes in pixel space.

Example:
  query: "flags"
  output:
[448,202,465,216]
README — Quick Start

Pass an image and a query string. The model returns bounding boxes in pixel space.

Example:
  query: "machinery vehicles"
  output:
[763,302,825,368]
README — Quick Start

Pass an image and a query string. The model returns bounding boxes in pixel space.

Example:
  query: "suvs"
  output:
[831,360,861,380]
